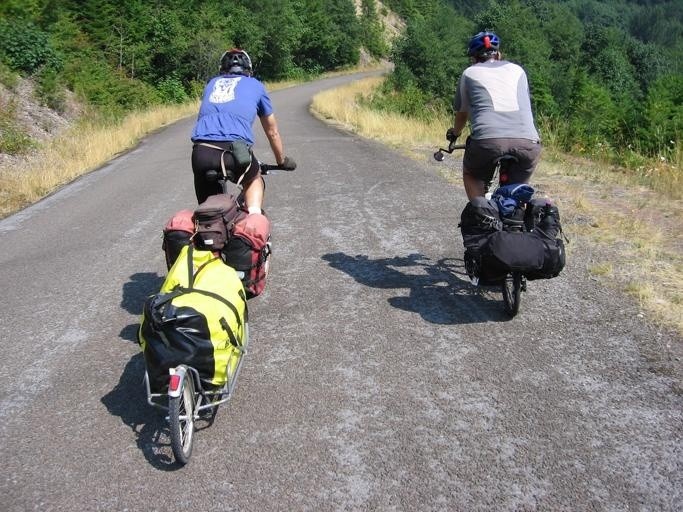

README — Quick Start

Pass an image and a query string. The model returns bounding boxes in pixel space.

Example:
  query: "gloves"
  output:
[446,128,458,141]
[278,157,295,170]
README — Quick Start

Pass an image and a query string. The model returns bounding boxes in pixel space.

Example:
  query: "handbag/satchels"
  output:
[162,209,196,272]
[230,141,250,168]
[460,184,565,281]
[221,214,270,300]
[135,242,249,396]
[191,194,239,250]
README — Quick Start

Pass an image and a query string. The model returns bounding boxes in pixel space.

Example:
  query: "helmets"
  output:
[466,32,499,57]
[218,50,253,76]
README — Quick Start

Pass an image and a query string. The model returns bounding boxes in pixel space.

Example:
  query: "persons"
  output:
[190,46,295,217]
[444,27,543,201]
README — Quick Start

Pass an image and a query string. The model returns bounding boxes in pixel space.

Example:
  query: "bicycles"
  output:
[191,160,291,304]
[433,131,527,316]
